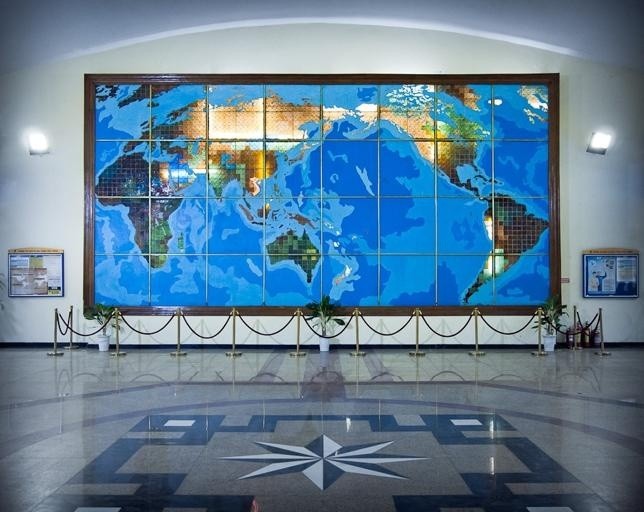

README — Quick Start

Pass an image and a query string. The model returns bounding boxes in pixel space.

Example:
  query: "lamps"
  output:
[587,132,612,155]
[27,130,50,156]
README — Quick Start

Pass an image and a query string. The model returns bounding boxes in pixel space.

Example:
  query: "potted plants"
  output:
[531,294,569,351]
[303,294,345,352]
[83,304,121,350]
